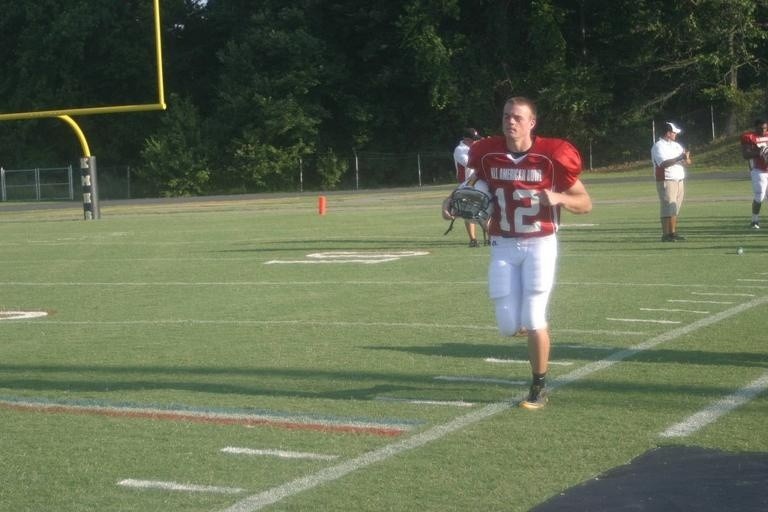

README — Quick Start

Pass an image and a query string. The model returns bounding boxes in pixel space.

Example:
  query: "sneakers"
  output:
[750,221,759,229]
[484,240,491,246]
[673,233,686,240]
[662,234,677,242]
[470,240,480,247]
[518,384,549,410]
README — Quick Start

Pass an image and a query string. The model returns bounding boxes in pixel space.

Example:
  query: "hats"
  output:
[662,121,681,134]
[464,128,481,140]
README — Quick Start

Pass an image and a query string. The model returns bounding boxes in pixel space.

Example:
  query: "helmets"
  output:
[449,179,493,224]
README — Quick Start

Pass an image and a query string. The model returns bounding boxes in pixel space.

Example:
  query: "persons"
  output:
[440,98,593,409]
[651,122,693,242]
[739,119,768,229]
[452,129,492,248]
